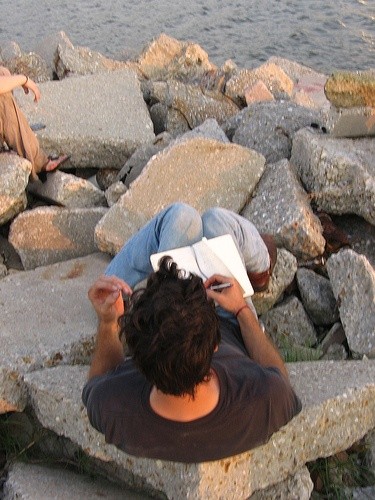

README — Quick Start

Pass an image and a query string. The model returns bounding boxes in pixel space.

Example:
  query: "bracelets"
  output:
[23,74,28,87]
[235,304,250,319]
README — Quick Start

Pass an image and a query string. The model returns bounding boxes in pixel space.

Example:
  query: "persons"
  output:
[79,201,303,464]
[0,65,70,184]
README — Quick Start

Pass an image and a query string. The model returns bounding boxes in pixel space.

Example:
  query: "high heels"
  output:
[36,155,71,185]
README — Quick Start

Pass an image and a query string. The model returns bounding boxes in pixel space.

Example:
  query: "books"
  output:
[149,233,256,309]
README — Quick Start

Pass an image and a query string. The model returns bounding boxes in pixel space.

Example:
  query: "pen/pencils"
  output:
[209,281,233,290]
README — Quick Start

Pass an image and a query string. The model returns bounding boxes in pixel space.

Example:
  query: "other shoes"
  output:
[247,233,278,291]
